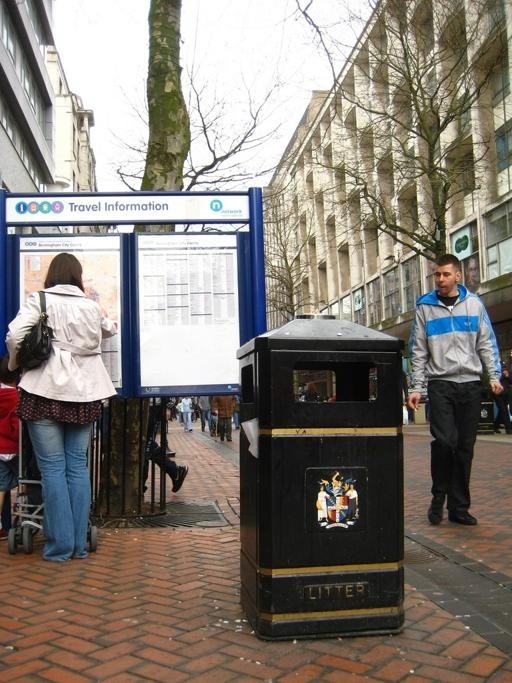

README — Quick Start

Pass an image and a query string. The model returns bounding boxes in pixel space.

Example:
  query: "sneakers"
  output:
[172,466,188,492]
[447,511,477,525]
[427,496,446,524]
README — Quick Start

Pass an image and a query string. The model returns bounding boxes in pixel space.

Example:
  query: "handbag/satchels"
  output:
[15,313,53,369]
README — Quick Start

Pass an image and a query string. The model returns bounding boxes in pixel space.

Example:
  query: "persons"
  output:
[4,250,120,558]
[406,253,506,525]
[143,394,242,492]
[297,362,511,437]
[0,352,22,541]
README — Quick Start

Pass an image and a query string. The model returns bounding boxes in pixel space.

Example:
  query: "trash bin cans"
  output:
[235,313,406,642]
[474,382,495,435]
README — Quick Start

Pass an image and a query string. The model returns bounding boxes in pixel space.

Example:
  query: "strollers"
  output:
[7,402,101,553]
